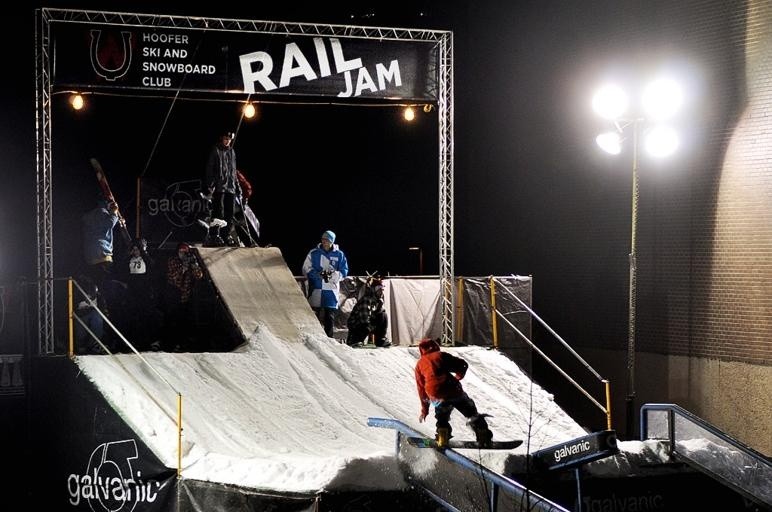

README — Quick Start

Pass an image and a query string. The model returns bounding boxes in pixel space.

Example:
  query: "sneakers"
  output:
[374,337,392,346]
[224,234,237,247]
[205,235,225,247]
[350,339,363,348]
[474,427,494,445]
[435,433,454,447]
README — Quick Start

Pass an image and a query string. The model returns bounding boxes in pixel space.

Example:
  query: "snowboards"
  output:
[90,158,132,245]
[407,437,523,450]
[235,196,261,237]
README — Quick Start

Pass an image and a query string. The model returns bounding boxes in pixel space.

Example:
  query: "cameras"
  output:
[320,271,332,283]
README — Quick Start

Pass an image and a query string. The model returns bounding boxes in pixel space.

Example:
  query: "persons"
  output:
[336,277,356,328]
[56,301,114,354]
[228,170,259,247]
[208,131,241,247]
[195,182,227,246]
[346,281,392,348]
[124,237,153,302]
[302,230,348,337]
[151,243,204,351]
[83,201,119,317]
[414,338,495,447]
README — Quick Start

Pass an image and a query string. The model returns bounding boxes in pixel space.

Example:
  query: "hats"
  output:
[320,230,337,243]
[218,129,236,140]
[175,241,191,256]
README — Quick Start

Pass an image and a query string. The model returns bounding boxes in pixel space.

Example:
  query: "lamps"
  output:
[402,103,416,121]
[68,87,84,111]
[239,100,255,118]
